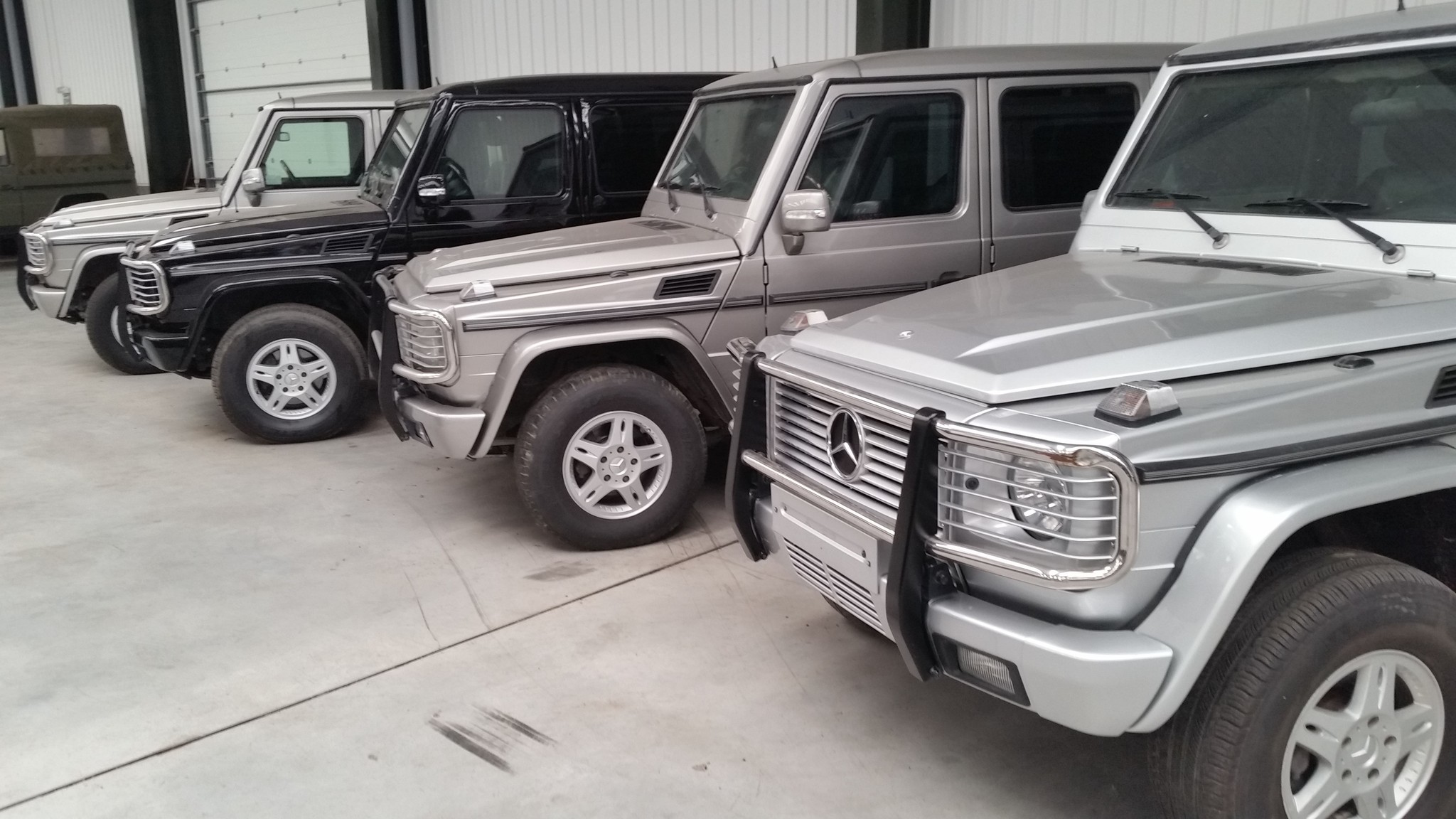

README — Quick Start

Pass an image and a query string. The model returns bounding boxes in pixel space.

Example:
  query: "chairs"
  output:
[1386,72,1456,177]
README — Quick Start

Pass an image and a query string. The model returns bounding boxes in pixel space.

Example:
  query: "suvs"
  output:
[16,89,435,374]
[371,44,1370,554]
[725,2,1455,819]
[117,72,722,443]
